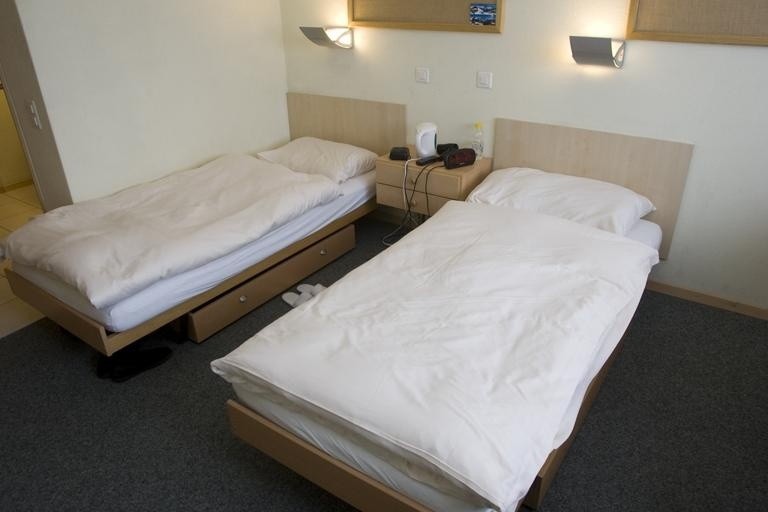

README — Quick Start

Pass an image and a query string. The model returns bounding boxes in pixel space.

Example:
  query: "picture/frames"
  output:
[623,2,768,47]
[347,0,504,33]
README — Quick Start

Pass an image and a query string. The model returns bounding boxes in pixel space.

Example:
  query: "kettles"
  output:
[415,123,438,157]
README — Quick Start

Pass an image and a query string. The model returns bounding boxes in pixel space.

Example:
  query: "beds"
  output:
[206,200,666,512]
[0,150,379,360]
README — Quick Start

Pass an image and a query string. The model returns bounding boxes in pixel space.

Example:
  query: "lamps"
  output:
[569,36,627,69]
[299,25,353,51]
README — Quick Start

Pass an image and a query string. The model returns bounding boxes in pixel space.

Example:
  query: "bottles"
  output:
[472,123,484,160]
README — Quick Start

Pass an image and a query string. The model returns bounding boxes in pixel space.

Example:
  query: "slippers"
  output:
[281,283,328,309]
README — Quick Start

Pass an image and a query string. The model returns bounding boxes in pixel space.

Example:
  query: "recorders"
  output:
[443,148,475,169]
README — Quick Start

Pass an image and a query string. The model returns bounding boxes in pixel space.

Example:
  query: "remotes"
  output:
[416,155,438,165]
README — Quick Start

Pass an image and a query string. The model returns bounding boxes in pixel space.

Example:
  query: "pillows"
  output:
[256,135,378,185]
[466,169,656,235]
